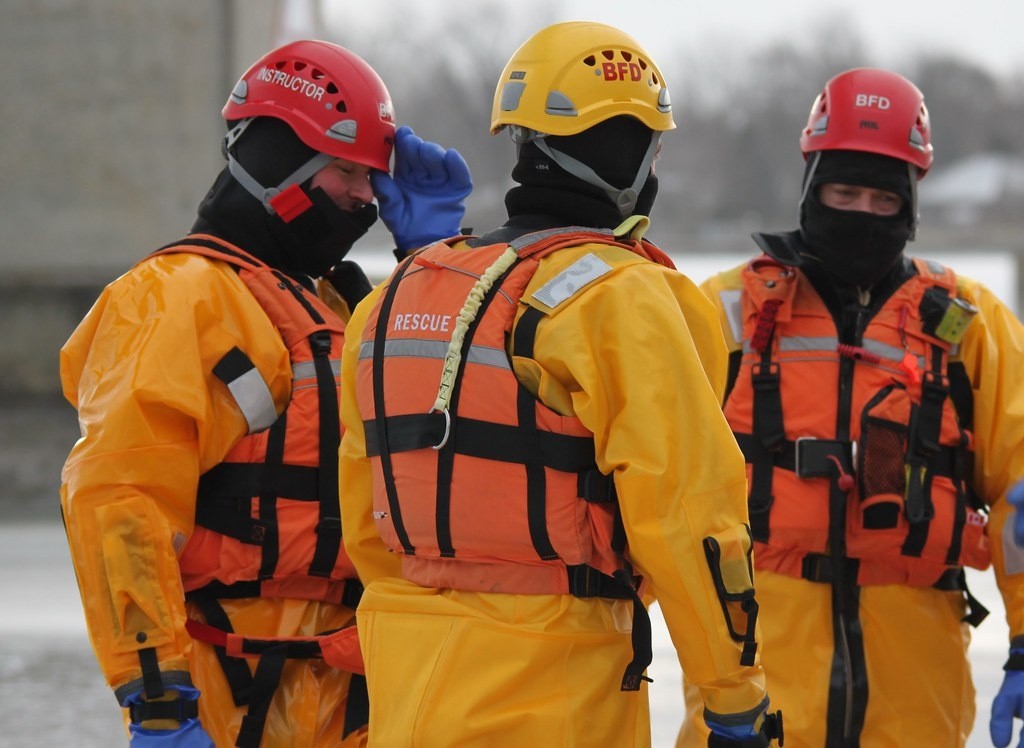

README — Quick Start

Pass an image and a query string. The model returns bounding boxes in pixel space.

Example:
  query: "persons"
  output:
[339,22,795,748]
[61,41,477,748]
[671,68,1023,748]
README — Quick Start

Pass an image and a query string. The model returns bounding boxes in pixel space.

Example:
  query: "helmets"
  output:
[488,20,677,136]
[221,39,396,173]
[799,68,935,169]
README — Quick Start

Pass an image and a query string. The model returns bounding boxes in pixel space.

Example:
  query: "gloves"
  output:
[989,635,1024,748]
[370,125,474,252]
[1005,480,1024,546]
[121,685,216,748]
